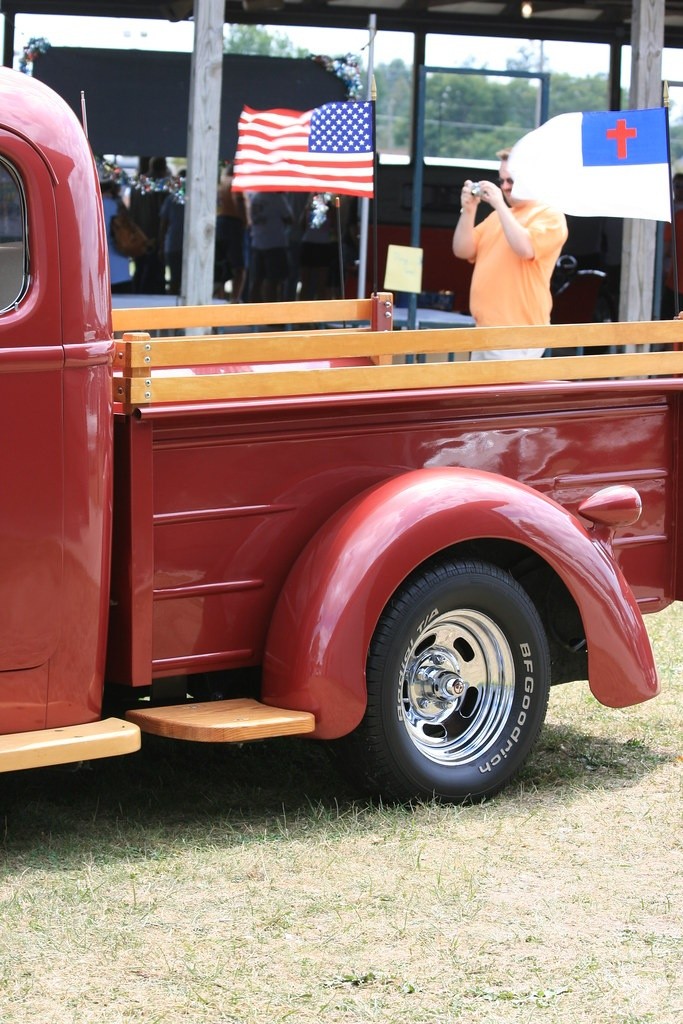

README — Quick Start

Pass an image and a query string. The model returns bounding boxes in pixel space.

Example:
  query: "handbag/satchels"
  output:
[110,210,148,257]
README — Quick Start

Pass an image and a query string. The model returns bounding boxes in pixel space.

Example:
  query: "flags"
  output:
[505,105,675,226]
[229,99,376,198]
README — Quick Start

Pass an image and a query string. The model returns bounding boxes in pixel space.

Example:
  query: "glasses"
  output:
[497,177,513,185]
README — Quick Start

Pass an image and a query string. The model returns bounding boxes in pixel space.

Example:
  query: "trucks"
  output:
[0,69,683,807]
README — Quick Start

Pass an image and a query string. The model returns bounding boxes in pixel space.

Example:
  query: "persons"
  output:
[662,172,683,319]
[452,149,569,364]
[93,153,351,335]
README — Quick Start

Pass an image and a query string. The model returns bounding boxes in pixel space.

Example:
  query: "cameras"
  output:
[468,183,484,196]
